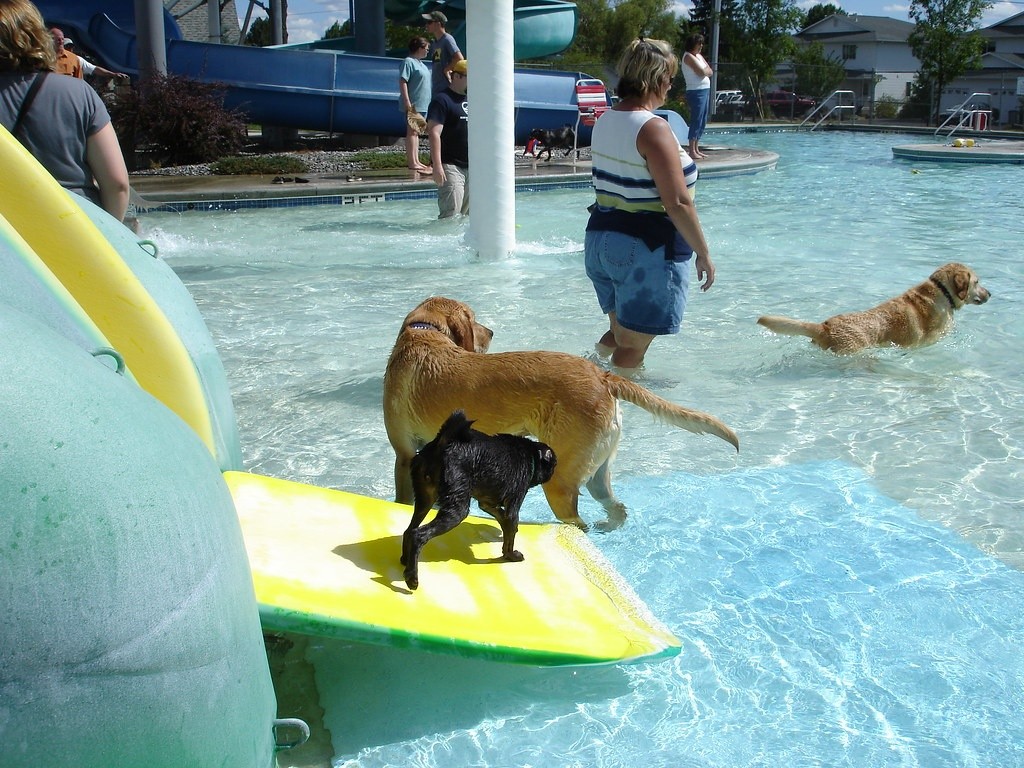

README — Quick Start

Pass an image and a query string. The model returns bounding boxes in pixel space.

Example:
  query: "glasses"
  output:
[425,19,439,24]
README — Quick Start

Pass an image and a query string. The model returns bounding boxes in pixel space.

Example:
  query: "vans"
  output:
[715,90,743,110]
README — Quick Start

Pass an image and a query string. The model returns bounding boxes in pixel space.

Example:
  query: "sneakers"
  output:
[346,174,363,182]
[271,177,284,184]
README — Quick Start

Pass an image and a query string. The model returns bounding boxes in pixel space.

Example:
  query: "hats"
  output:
[421,11,447,25]
[62,37,73,46]
[452,60,468,72]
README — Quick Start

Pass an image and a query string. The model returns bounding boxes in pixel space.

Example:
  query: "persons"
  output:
[0,0,131,224]
[579,37,716,382]
[426,58,468,220]
[680,32,714,160]
[416,9,464,177]
[48,27,84,81]
[398,36,432,170]
[61,38,130,80]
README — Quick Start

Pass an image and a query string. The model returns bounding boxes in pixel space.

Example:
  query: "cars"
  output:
[764,91,816,118]
[938,102,994,126]
[721,94,753,118]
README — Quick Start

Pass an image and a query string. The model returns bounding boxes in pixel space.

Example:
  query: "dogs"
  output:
[399,410,559,592]
[951,138,975,147]
[380,294,738,535]
[755,262,992,357]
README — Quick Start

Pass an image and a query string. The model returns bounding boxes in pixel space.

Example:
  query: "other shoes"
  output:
[295,176,310,183]
[282,176,292,182]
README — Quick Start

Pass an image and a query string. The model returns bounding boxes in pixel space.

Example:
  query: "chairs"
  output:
[571,78,611,165]
